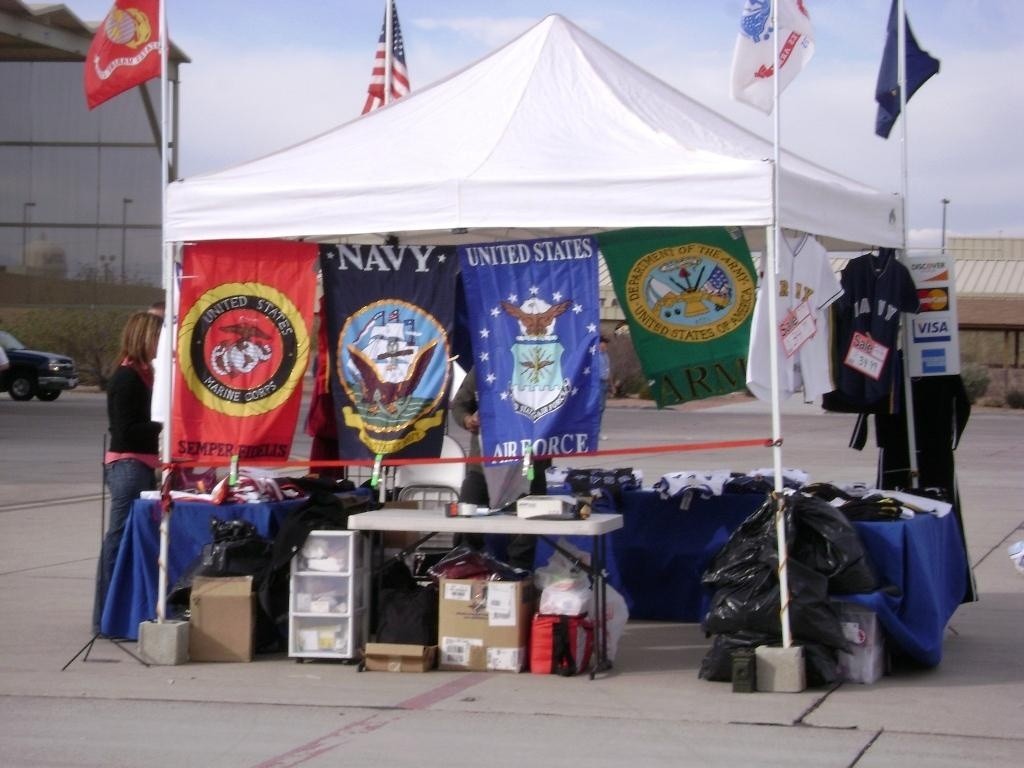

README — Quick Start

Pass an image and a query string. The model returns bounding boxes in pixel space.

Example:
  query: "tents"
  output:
[152,11,920,650]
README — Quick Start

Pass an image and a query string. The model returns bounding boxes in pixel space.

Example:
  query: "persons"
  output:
[0,343,12,372]
[598,335,617,441]
[449,365,552,575]
[91,301,166,639]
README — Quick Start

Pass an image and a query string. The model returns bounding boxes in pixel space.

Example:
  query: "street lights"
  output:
[101,254,116,282]
[121,199,133,274]
[942,198,950,254]
[23,202,36,264]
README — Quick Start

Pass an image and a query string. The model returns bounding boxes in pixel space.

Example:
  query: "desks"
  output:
[347,505,625,685]
[97,493,968,665]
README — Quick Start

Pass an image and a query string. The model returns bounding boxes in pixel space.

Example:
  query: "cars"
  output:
[0,329,80,401]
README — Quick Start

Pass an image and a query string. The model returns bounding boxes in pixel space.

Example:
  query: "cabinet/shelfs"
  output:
[288,530,367,663]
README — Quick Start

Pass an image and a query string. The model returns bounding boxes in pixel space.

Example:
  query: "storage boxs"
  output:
[515,495,578,519]
[363,642,438,673]
[833,604,888,684]
[187,575,257,662]
[437,575,536,671]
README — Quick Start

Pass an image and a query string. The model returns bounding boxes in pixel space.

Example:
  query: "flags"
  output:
[730,1,815,116]
[316,243,462,460]
[359,0,413,117]
[456,234,606,466]
[875,0,942,141]
[83,0,165,112]
[169,241,320,460]
[596,227,759,410]
